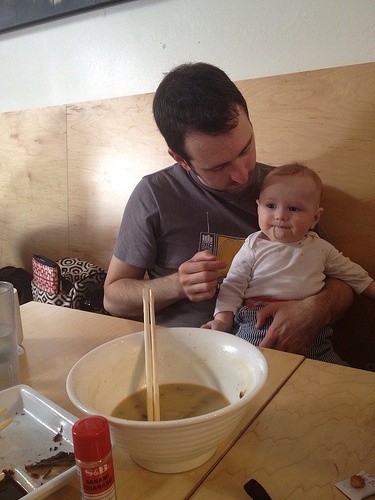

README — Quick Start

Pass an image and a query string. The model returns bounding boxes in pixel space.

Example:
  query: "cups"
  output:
[0,280,20,391]
[13,288,24,345]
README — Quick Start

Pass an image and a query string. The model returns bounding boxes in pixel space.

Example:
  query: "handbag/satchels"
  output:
[30,254,110,315]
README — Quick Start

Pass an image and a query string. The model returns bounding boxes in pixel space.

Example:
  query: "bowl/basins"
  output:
[66,327,268,473]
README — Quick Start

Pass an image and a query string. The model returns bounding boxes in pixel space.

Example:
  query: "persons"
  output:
[200,164,375,367]
[103,62,355,356]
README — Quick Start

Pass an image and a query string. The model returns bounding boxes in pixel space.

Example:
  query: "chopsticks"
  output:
[143,288,160,422]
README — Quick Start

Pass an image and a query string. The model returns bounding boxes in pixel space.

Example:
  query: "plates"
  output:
[0,384,81,500]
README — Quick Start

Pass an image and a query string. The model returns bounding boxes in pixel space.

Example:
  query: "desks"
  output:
[20,300,375,500]
